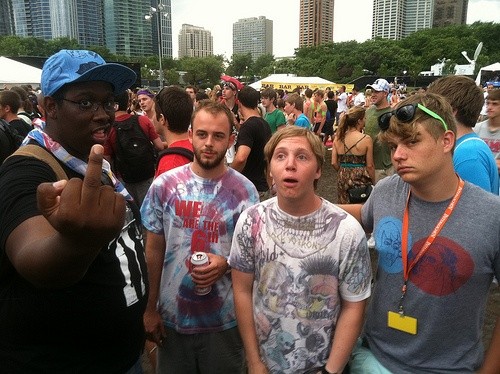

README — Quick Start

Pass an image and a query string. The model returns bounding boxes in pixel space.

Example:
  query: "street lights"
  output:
[145,3,170,91]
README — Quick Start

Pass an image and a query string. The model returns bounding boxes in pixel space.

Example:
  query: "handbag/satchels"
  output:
[347,182,374,204]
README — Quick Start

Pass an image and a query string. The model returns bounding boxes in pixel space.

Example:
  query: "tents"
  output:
[248,73,337,92]
[474,62,500,86]
[0,56,43,90]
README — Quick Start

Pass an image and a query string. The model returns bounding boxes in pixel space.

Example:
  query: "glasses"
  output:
[378,103,448,132]
[55,97,120,112]
[153,87,166,118]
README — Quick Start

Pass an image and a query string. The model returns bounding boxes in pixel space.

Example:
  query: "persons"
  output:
[0,50,152,374]
[140,100,260,374]
[361,79,393,187]
[128,79,428,160]
[477,84,493,122]
[227,123,372,374]
[153,84,195,181]
[229,87,273,203]
[334,93,500,374]
[104,90,167,208]
[0,85,45,164]
[332,106,375,204]
[426,75,500,195]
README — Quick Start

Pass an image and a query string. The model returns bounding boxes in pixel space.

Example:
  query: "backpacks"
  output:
[112,116,155,183]
[0,119,25,155]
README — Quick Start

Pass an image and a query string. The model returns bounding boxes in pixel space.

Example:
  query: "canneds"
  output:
[190,251,211,295]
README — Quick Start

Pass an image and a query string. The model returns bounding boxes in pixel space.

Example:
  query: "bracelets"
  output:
[321,363,338,374]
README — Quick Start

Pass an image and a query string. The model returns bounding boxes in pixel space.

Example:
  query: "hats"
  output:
[365,79,391,95]
[40,50,137,97]
